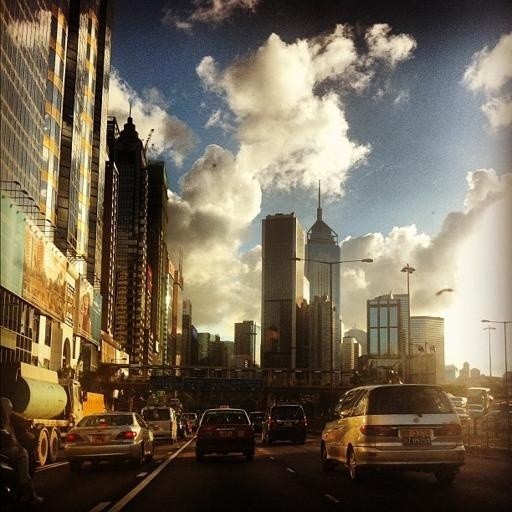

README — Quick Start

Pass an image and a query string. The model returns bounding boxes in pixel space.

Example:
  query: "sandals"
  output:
[464,387,494,410]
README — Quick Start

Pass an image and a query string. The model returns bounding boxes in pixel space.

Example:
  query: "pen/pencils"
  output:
[320,382,468,488]
[141,407,178,447]
[261,403,307,448]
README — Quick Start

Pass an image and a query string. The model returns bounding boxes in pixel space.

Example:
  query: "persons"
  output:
[1,394,44,507]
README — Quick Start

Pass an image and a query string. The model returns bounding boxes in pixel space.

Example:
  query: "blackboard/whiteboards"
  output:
[0,361,89,469]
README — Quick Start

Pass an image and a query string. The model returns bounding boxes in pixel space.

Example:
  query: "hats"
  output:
[448,397,512,438]
[249,411,265,433]
[64,411,156,475]
[175,412,199,439]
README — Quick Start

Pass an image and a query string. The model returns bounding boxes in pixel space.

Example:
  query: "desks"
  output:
[290,257,374,420]
[401,263,416,383]
[111,357,128,365]
[484,327,497,378]
[481,320,512,412]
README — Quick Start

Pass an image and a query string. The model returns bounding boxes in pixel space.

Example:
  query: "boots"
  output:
[194,408,256,462]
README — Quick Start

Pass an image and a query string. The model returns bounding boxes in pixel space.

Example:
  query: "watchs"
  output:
[0,424,44,506]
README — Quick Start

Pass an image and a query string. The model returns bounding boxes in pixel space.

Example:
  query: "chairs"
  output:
[18,489,45,504]
